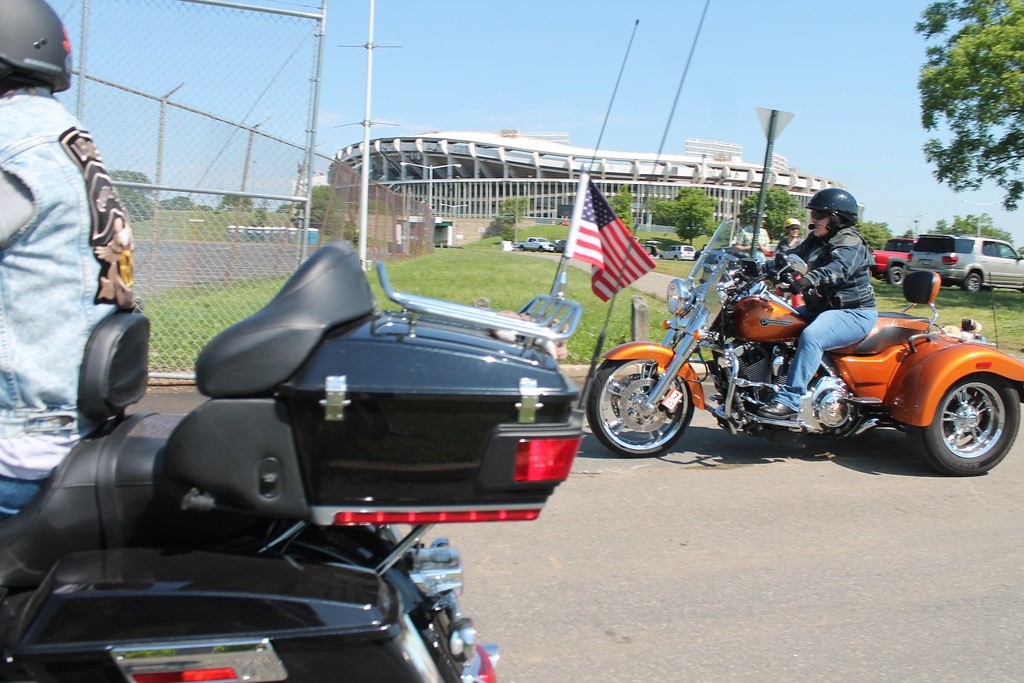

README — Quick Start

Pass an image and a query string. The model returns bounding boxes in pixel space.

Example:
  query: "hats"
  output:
[748,208,768,218]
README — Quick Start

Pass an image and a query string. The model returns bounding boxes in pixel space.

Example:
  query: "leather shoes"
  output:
[756,400,798,422]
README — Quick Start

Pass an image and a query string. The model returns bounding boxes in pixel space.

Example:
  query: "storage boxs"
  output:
[0,549,446,683]
[276,309,586,509]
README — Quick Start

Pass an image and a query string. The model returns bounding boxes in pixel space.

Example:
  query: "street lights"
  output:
[440,203,469,243]
[401,161,462,209]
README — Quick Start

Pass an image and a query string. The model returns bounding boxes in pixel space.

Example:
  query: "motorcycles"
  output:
[583,214,1023,477]
[0,242,584,683]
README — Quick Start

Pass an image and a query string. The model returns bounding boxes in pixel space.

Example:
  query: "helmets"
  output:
[783,218,801,230]
[0,0,71,97]
[804,188,858,224]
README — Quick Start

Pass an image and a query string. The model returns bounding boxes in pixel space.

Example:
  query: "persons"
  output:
[0,0,134,523]
[775,219,805,255]
[731,210,772,302]
[757,189,878,422]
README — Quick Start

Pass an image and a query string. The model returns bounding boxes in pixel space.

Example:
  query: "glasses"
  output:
[790,224,799,230]
[811,209,831,220]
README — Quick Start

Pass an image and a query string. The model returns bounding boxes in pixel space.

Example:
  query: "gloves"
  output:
[787,277,813,295]
[761,259,775,275]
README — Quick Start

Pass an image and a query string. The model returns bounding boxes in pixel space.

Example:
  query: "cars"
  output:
[553,240,566,252]
[642,245,659,258]
[763,244,778,259]
[698,245,733,262]
[659,245,696,261]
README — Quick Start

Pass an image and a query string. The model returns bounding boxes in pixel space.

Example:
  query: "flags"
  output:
[563,173,656,302]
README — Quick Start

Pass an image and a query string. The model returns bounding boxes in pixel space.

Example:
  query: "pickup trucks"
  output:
[515,237,556,253]
[871,237,917,287]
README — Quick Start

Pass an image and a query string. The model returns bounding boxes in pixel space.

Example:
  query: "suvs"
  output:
[906,233,1023,292]
